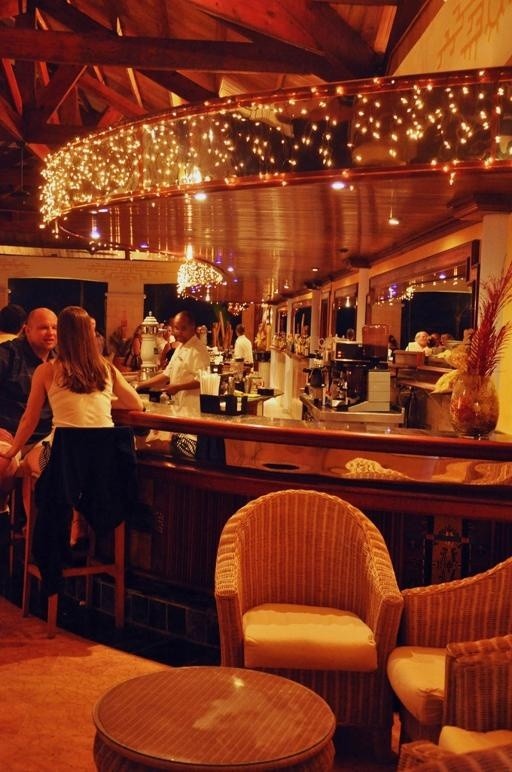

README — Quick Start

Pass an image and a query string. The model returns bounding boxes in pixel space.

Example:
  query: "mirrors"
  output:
[274,239,478,368]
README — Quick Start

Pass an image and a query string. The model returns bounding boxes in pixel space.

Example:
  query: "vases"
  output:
[449,371,499,441]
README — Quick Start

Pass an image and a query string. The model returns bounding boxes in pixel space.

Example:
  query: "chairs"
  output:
[20,427,136,636]
[386,554,512,772]
[214,489,405,767]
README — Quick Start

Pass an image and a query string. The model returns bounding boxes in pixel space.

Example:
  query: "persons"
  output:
[232,324,253,394]
[290,325,310,352]
[388,328,473,362]
[131,311,210,409]
[0,302,114,462]
[346,328,355,341]
[0,306,143,603]
